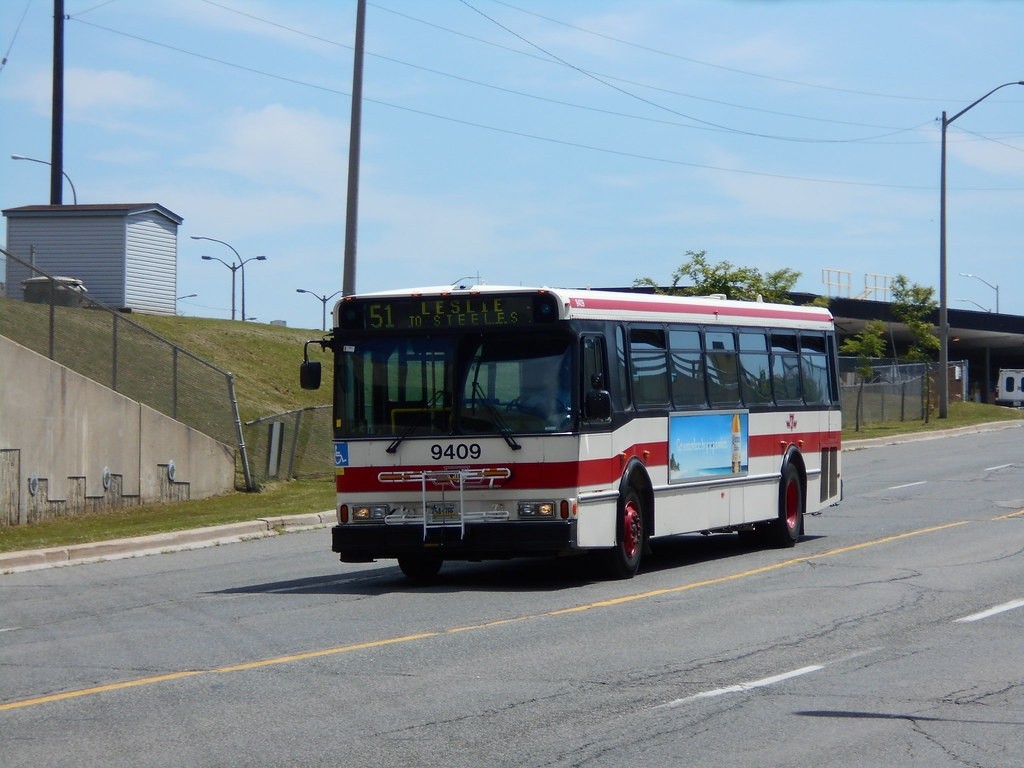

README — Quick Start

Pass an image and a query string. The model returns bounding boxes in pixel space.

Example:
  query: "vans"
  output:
[994,369,1024,408]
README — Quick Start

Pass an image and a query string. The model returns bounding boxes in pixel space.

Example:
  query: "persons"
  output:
[521,367,571,424]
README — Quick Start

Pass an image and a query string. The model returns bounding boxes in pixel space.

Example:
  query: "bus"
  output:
[299,283,844,584]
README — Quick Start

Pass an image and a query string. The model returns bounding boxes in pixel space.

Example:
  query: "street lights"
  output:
[296,286,343,330]
[190,234,246,320]
[11,154,77,205]
[201,255,267,320]
[959,273,1000,314]
